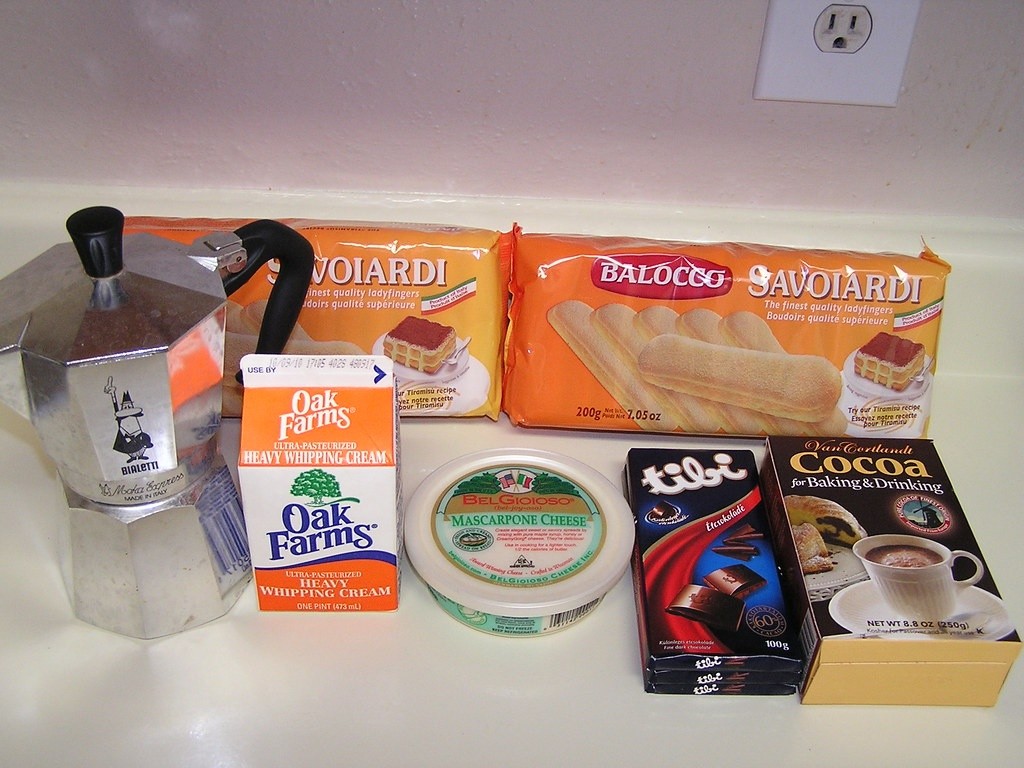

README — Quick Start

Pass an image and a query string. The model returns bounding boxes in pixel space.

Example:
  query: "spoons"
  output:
[823,611,993,640]
[909,353,933,382]
[441,336,472,365]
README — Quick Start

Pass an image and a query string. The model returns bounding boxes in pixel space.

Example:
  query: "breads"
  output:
[783,494,869,575]
[219,299,368,416]
[549,300,848,439]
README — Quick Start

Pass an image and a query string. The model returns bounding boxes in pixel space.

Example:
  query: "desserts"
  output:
[854,331,925,391]
[384,316,457,375]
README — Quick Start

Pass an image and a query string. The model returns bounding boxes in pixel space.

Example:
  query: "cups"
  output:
[852,534,985,622]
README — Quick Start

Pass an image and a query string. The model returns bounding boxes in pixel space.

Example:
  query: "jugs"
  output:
[1,206,314,640]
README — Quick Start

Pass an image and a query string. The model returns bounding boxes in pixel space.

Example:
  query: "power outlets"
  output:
[753,0,921,107]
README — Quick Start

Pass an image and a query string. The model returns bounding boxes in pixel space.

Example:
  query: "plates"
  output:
[829,579,1016,640]
[372,332,471,382]
[843,347,931,401]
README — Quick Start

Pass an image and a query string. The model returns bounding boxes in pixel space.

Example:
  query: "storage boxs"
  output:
[621,447,809,695]
[237,350,404,613]
[758,436,1023,706]
